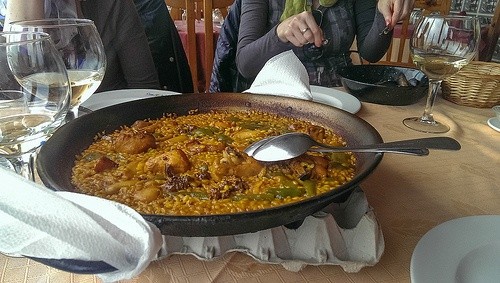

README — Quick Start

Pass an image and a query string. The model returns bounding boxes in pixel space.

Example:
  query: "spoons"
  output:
[252,132,462,162]
[244,136,430,161]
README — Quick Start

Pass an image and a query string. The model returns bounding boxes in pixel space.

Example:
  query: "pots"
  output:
[22,92,384,274]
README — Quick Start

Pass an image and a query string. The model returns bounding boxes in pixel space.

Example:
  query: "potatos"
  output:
[91,118,327,204]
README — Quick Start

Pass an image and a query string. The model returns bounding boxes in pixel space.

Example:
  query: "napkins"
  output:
[0,166,163,283]
[249,50,314,102]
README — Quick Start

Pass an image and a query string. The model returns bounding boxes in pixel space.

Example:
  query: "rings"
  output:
[301,27,310,35]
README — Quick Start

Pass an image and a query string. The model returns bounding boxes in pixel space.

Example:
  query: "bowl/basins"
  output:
[335,65,429,106]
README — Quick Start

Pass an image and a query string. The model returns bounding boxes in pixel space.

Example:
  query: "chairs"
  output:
[164,0,452,94]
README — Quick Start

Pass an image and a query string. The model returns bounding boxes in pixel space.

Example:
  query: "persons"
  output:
[236,0,417,89]
[0,0,161,100]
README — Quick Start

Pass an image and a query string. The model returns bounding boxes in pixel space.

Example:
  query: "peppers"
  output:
[186,117,353,203]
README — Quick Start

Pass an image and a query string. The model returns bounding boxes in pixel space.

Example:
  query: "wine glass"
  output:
[402,14,481,134]
[0,31,72,181]
[6,18,107,124]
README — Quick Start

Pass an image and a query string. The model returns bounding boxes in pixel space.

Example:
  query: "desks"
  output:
[0,83,500,283]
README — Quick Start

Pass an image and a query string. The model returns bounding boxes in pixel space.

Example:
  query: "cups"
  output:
[0,90,28,141]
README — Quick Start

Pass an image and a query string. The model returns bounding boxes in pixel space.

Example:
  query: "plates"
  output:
[78,89,182,119]
[410,215,500,283]
[241,85,361,114]
[487,117,500,132]
